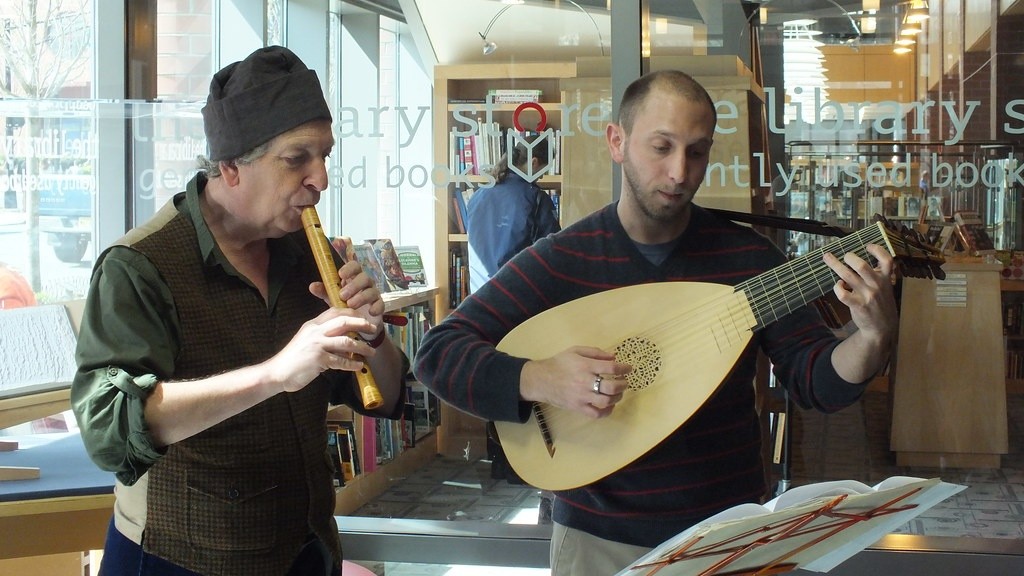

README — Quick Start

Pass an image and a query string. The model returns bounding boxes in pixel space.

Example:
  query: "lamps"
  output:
[478,0,604,56]
[893,0,931,53]
[862,0,881,11]
[861,17,876,34]
[783,19,831,119]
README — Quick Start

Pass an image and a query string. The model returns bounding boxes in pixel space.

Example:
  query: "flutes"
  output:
[300,206,385,410]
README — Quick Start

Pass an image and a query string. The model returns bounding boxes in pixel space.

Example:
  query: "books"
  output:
[452,186,485,233]
[448,87,545,104]
[448,117,561,176]
[827,182,944,235]
[999,300,1024,336]
[924,224,952,254]
[354,302,443,476]
[323,416,362,490]
[539,188,564,230]
[1004,348,1024,381]
[955,210,997,257]
[449,248,471,308]
[327,234,429,295]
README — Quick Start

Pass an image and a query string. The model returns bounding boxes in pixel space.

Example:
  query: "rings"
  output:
[592,376,605,395]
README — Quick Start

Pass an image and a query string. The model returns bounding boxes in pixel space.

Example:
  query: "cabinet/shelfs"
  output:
[335,288,443,514]
[886,259,1010,468]
[0,409,116,559]
[434,53,786,475]
[788,159,930,253]
[1001,280,1024,396]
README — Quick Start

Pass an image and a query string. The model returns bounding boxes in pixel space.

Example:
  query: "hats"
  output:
[200,45,332,163]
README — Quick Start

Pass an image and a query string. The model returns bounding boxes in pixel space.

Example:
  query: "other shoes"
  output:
[504,466,524,484]
[490,460,507,479]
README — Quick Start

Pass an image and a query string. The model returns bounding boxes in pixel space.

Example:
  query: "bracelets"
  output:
[359,314,409,349]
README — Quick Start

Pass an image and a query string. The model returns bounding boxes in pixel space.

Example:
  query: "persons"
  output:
[67,43,412,576]
[465,136,562,294]
[0,263,34,311]
[411,68,900,575]
